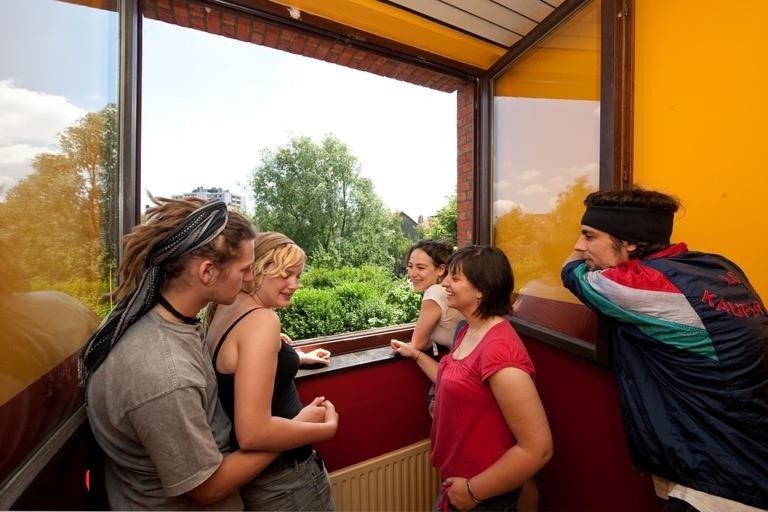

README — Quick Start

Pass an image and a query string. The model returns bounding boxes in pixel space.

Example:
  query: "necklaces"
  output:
[158,295,200,325]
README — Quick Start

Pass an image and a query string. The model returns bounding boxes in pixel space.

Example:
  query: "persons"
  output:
[203,231,342,512]
[390,246,554,512]
[76,194,326,512]
[561,187,767,512]
[405,238,454,418]
[519,238,583,307]
[0,240,106,403]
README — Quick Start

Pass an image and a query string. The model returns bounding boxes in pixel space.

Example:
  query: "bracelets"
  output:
[415,351,422,362]
[466,479,483,504]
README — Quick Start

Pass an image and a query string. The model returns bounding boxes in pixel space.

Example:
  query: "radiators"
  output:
[325,436,443,512]
